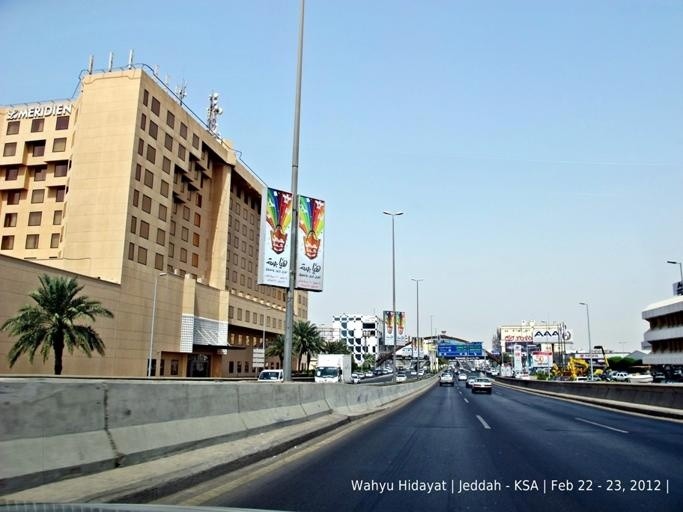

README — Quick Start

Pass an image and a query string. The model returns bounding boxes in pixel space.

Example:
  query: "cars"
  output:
[256,368,284,383]
[465,375,478,387]
[458,372,467,381]
[514,370,530,380]
[471,377,492,394]
[439,372,454,386]
[351,365,427,384]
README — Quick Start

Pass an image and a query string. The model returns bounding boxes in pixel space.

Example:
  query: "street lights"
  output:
[382,209,404,383]
[580,302,595,381]
[667,259,682,285]
[146,271,168,377]
[410,275,425,381]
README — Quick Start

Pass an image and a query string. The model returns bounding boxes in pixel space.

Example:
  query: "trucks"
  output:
[313,352,353,384]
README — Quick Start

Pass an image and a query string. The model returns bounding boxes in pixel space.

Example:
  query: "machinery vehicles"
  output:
[593,344,610,376]
[551,356,589,381]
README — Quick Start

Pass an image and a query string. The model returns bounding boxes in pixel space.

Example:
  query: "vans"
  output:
[608,370,629,382]
